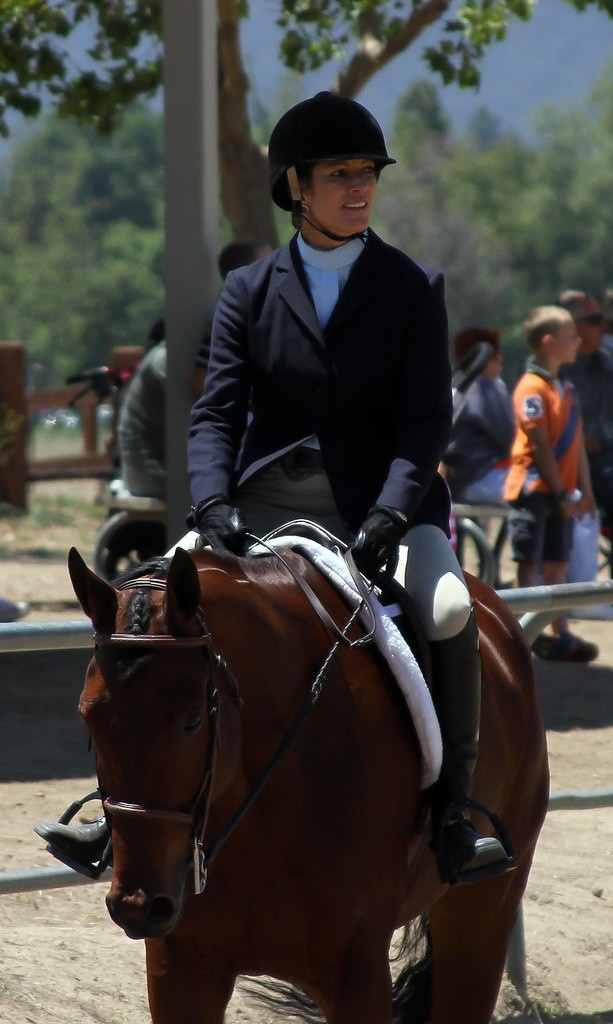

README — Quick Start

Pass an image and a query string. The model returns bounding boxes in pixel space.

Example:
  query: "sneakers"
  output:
[534,630,599,665]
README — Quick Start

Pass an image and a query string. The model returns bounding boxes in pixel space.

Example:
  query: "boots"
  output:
[417,608,507,885]
[33,813,113,870]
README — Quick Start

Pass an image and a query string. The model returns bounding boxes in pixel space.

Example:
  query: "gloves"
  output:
[350,506,410,585]
[195,501,250,559]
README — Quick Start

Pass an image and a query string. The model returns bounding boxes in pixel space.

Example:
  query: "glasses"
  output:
[573,313,606,327]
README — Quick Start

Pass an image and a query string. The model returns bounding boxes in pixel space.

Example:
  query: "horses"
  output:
[66,539,550,1024]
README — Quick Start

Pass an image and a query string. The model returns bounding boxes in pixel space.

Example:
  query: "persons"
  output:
[34,91,508,883]
[443,286,613,663]
[116,318,169,501]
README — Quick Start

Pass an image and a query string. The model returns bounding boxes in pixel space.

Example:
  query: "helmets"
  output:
[268,91,398,213]
